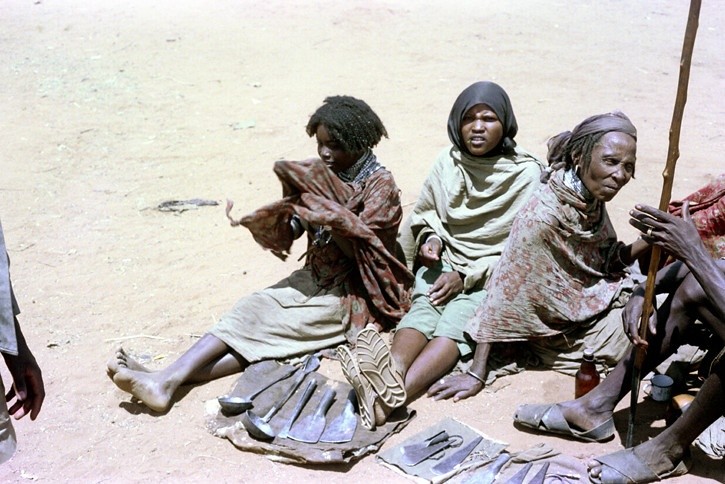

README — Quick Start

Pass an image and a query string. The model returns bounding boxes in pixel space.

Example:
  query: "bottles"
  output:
[575,349,600,399]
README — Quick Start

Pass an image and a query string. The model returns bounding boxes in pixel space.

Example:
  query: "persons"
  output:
[426,113,725,484]
[335,81,550,431]
[103,96,416,411]
[0,221,45,466]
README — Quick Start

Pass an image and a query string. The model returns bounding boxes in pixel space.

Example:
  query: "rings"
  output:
[440,379,444,384]
[646,227,652,238]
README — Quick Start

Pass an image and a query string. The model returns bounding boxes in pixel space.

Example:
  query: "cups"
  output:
[650,375,674,401]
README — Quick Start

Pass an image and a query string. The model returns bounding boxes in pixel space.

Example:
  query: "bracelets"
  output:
[426,235,443,250]
[467,368,486,383]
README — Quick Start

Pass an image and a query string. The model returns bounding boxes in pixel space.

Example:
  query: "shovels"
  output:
[319,389,359,443]
[288,389,336,443]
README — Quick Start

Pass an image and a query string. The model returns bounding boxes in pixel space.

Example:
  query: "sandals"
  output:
[513,402,616,442]
[587,439,692,484]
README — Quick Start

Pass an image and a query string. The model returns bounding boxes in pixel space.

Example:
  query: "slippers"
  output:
[336,345,379,432]
[355,328,407,408]
